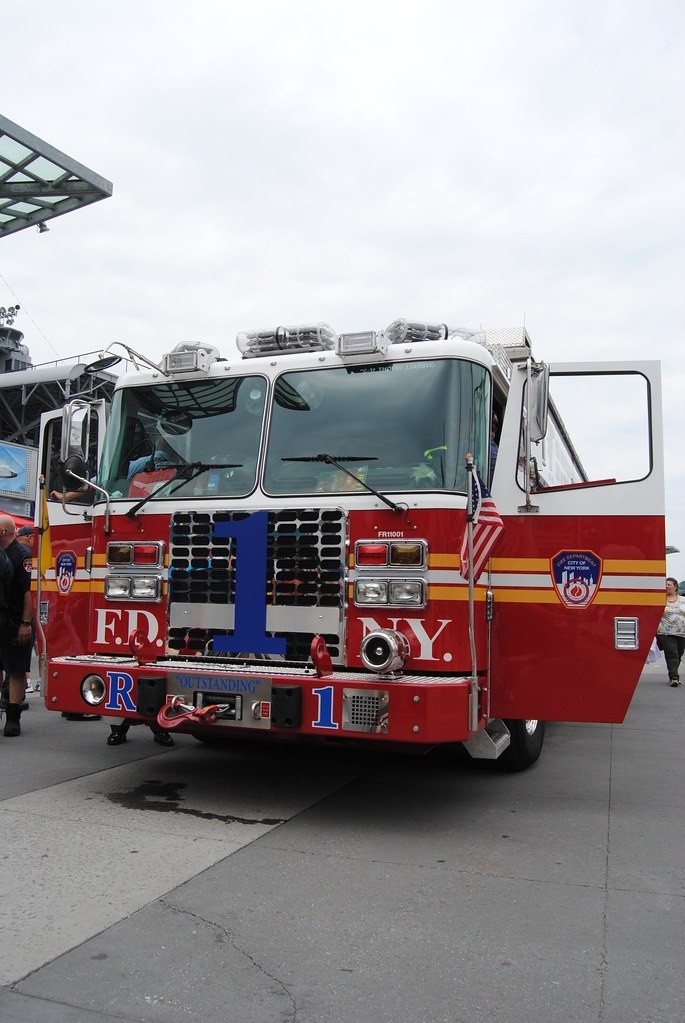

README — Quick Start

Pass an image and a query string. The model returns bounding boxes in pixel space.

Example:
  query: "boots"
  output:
[3,703,22,736]
[0,680,29,710]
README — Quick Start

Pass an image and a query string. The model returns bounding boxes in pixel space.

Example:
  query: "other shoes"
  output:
[671,678,681,687]
[34,682,40,691]
[25,681,33,693]
[66,713,101,721]
[61,712,66,717]
[107,731,126,745]
[154,733,175,746]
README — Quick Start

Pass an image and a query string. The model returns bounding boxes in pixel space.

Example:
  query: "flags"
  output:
[459,465,506,584]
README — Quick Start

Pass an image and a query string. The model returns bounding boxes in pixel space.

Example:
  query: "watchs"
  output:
[21,619,32,626]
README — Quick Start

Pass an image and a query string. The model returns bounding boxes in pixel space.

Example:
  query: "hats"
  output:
[17,526,33,535]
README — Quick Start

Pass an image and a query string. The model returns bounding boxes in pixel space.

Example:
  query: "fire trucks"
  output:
[21,316,668,774]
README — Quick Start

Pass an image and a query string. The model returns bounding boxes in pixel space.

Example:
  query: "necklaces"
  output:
[667,595,678,602]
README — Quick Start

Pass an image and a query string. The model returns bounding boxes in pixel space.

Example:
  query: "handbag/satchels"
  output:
[657,637,663,651]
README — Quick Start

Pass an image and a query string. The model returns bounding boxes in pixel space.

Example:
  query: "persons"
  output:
[0,514,103,737]
[457,410,498,493]
[107,719,176,748]
[52,436,189,502]
[655,578,685,687]
